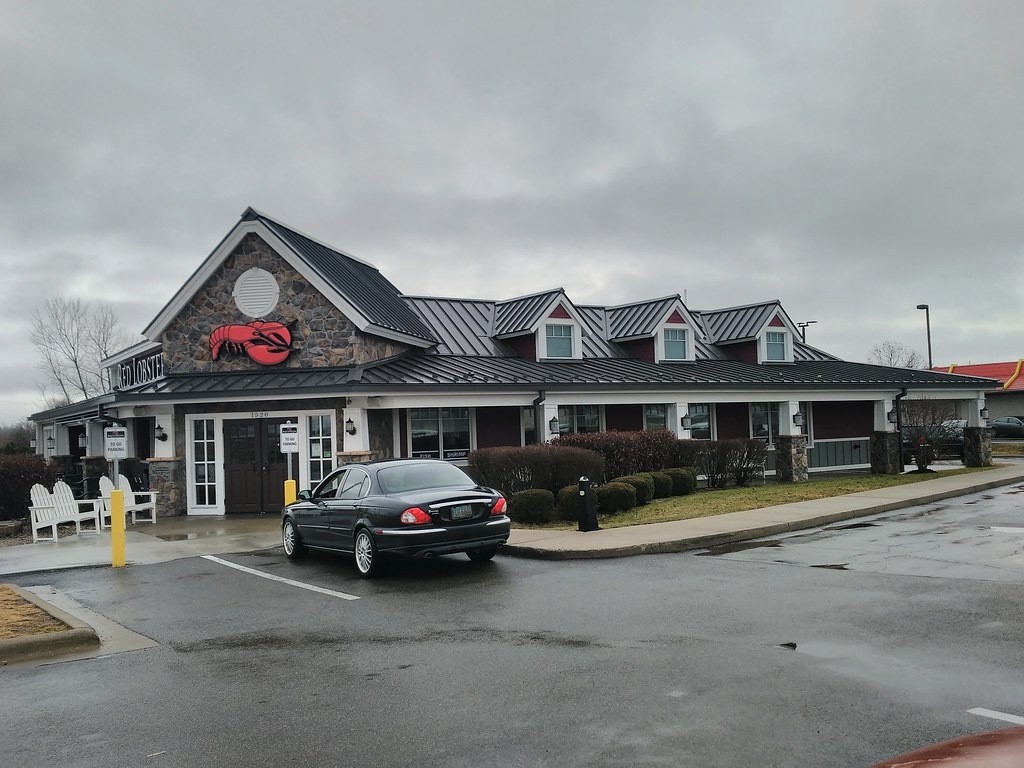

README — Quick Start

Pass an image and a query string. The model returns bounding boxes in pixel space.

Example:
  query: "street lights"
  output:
[917,303,936,371]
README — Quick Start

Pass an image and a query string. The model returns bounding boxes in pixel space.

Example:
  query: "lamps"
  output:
[887,409,897,423]
[681,412,691,430]
[79,432,88,449]
[47,436,55,449]
[29,438,36,449]
[793,411,803,426]
[345,418,357,435]
[155,424,168,442]
[549,416,560,435]
[980,406,989,419]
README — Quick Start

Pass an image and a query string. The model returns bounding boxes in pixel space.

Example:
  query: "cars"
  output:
[985,416,1024,439]
[280,458,511,578]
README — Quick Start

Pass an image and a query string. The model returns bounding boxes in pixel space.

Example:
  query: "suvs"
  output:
[900,424,966,464]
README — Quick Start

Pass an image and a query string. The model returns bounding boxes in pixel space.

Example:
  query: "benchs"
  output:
[28,480,101,543]
[97,474,159,530]
